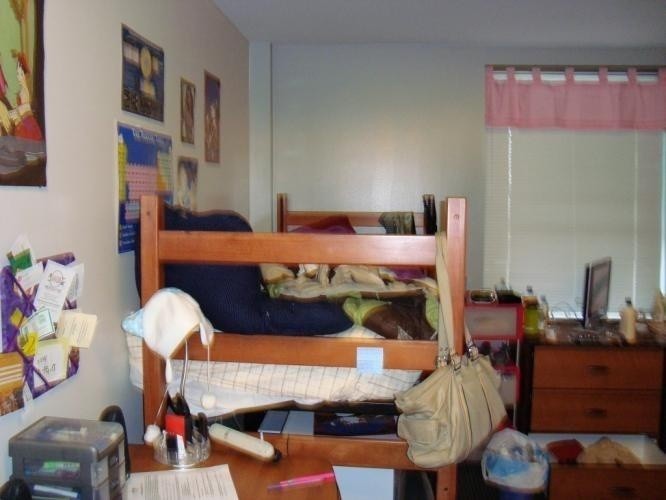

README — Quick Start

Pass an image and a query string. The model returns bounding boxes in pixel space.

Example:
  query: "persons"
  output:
[204,97,219,162]
[183,86,194,143]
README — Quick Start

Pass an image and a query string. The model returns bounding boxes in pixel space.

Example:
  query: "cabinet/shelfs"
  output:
[519,326,664,444]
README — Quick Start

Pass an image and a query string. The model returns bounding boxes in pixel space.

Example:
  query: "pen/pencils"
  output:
[266,472,335,492]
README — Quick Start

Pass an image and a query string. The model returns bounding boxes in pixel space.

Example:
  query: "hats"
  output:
[121,288,214,446]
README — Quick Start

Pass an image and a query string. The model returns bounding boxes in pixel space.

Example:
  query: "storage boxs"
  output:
[6,416,127,500]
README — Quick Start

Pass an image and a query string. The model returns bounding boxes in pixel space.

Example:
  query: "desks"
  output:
[123,436,336,498]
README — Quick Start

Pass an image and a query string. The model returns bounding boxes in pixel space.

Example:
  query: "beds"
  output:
[138,190,468,498]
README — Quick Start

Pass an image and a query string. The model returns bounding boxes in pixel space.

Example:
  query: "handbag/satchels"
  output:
[395,346,507,468]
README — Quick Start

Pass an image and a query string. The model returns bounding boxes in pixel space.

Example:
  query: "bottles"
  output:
[619,298,638,345]
[523,285,549,336]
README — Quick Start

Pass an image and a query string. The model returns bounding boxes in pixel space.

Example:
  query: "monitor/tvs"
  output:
[573,256,615,335]
